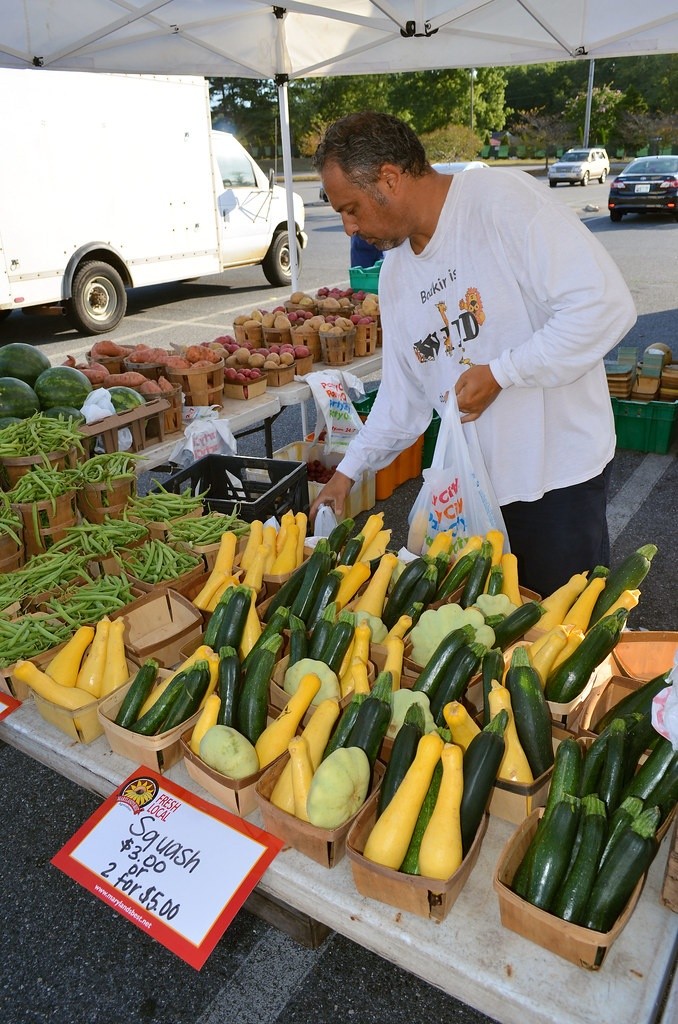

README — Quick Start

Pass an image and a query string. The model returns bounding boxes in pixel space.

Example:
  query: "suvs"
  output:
[548,147,610,188]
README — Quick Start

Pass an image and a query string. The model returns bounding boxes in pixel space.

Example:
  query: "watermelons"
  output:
[34,365,92,410]
[0,343,51,386]
[0,417,23,430]
[0,377,40,418]
[106,386,148,430]
[44,406,86,425]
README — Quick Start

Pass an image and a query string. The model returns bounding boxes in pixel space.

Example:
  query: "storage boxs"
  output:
[0,259,678,968]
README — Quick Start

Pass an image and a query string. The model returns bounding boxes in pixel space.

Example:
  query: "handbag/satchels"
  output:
[407,384,510,562]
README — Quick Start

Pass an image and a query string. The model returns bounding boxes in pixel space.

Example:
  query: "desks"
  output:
[137,396,281,477]
[265,345,383,457]
[0,702,678,1024]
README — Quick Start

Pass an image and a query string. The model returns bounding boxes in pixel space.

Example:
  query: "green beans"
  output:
[0,411,252,667]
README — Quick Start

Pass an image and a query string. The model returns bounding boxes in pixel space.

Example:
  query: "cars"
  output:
[432,161,491,173]
[608,155,678,223]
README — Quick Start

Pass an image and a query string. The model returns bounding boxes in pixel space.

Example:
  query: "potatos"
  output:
[61,291,379,405]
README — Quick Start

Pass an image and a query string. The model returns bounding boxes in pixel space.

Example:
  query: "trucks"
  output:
[0,68,307,334]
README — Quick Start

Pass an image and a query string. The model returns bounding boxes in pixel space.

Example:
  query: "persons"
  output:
[309,111,638,596]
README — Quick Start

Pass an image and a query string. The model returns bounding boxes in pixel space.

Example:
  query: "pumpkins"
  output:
[386,689,438,740]
[200,724,260,781]
[410,604,496,667]
[284,658,342,706]
[307,746,370,830]
[471,594,518,617]
[356,611,388,644]
